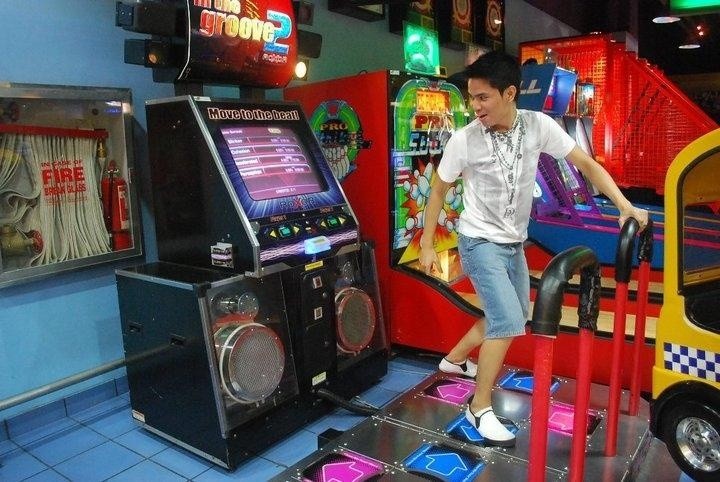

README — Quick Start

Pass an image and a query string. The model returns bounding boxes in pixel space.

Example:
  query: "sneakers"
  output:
[466,403,516,447]
[438,356,478,377]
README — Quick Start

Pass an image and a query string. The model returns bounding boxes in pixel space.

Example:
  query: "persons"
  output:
[419,49,648,447]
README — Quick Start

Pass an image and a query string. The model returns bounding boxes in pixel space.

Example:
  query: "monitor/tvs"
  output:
[216,118,333,202]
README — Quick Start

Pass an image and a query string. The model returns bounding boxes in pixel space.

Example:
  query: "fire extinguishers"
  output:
[102,160,130,250]
